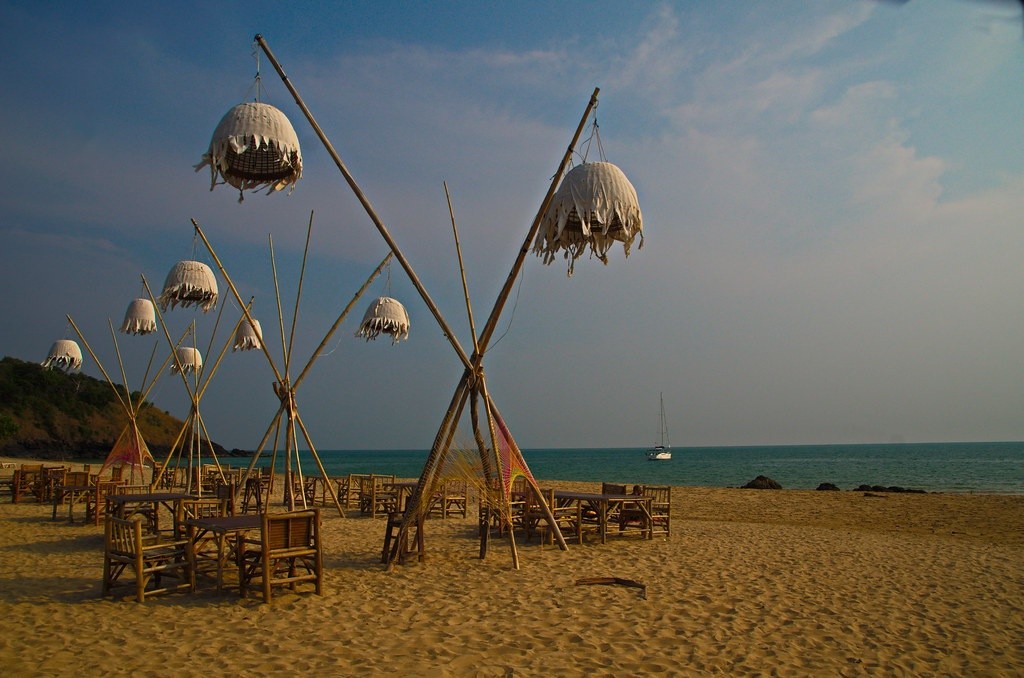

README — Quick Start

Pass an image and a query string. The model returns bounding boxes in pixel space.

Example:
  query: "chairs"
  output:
[479,484,526,536]
[2,462,128,525]
[360,474,396,507]
[443,476,469,519]
[257,467,274,494]
[238,467,261,494]
[342,473,373,509]
[114,483,159,537]
[183,485,237,522]
[178,499,246,576]
[240,508,322,604]
[360,476,400,518]
[525,487,582,546]
[577,483,627,524]
[186,464,222,491]
[284,471,315,505]
[495,478,527,531]
[152,461,179,490]
[103,512,195,603]
[618,486,672,540]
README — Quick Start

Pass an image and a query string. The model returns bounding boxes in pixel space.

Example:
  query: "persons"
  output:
[625,485,645,520]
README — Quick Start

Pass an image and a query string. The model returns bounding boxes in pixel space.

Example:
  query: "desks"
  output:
[184,512,299,598]
[382,482,438,519]
[168,465,204,490]
[106,494,196,538]
[0,475,26,503]
[304,475,366,507]
[56,485,113,523]
[209,469,254,498]
[510,490,656,544]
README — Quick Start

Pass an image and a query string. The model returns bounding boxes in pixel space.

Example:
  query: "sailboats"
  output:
[648,391,673,460]
